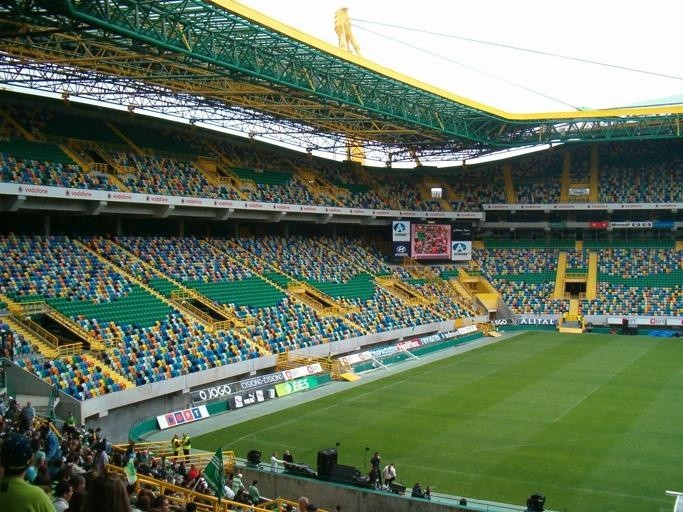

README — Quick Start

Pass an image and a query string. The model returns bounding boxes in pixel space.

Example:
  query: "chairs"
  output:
[0,139,683,403]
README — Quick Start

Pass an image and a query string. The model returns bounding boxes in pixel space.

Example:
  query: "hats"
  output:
[1,439,33,469]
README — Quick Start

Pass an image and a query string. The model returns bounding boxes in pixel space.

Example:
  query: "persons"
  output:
[368,452,382,488]
[382,464,396,488]
[411,482,423,497]
[0,398,346,511]
[413,225,448,255]
[421,486,431,499]
